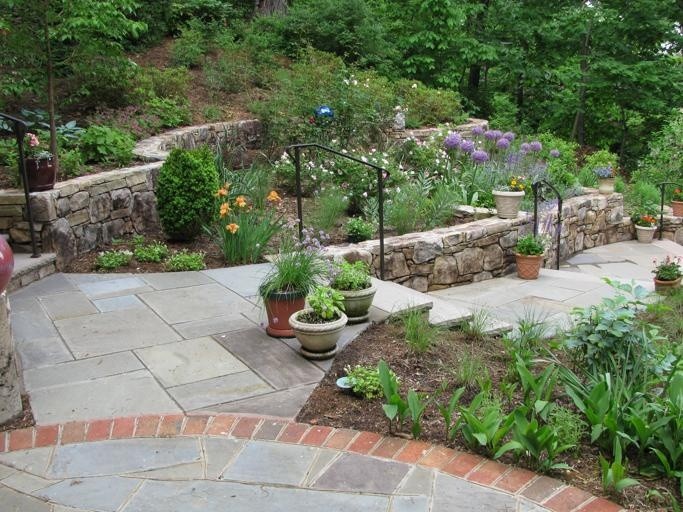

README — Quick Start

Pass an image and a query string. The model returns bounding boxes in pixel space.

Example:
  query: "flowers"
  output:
[592,161,617,178]
[23,132,53,172]
[671,188,683,201]
[493,174,527,192]
[649,253,683,281]
[245,215,341,317]
[634,210,657,228]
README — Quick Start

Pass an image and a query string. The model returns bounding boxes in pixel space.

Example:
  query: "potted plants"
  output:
[289,259,377,360]
[513,233,551,284]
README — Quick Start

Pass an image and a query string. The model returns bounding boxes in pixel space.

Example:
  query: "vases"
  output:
[671,201,683,217]
[491,190,525,219]
[24,155,60,193]
[634,224,657,244]
[652,276,682,296]
[258,285,309,339]
[598,177,615,195]
